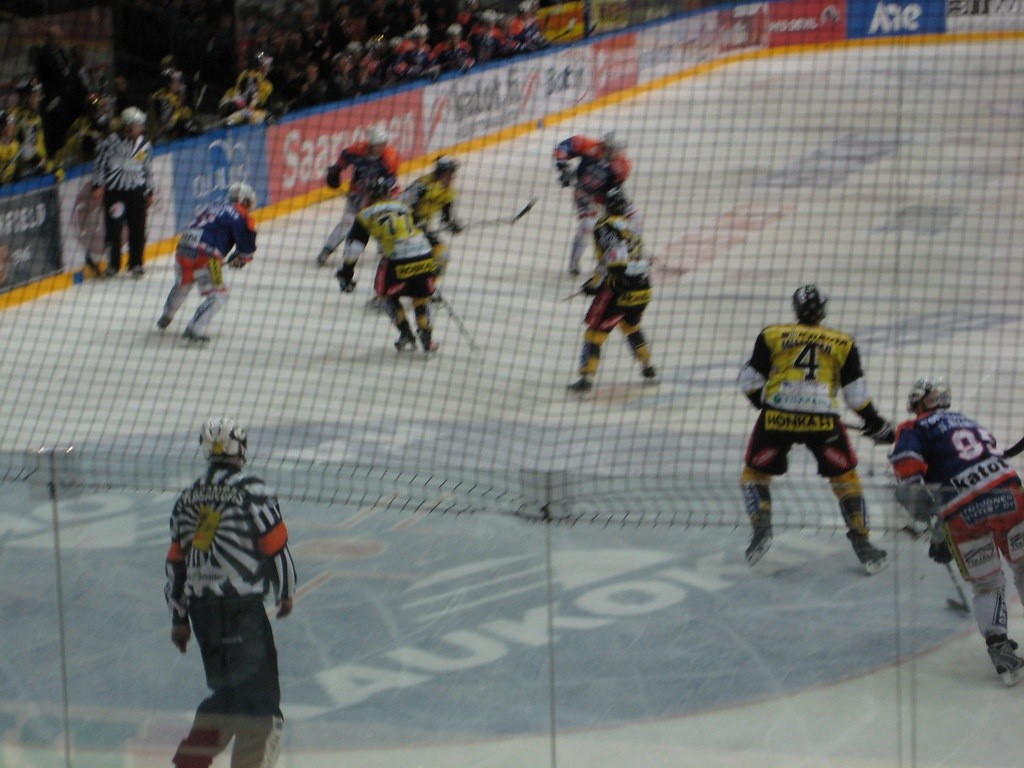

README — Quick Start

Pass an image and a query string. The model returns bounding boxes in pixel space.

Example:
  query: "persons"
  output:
[163,416,298,768]
[565,187,659,399]
[737,285,897,578]
[889,374,1024,686]
[0,0,551,185]
[158,183,258,348]
[551,129,645,276]
[334,175,444,358]
[317,130,404,292]
[92,107,157,280]
[371,154,462,304]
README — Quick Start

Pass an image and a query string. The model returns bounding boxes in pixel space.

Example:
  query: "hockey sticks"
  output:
[425,197,538,238]
[570,180,698,273]
[560,289,585,305]
[130,261,230,278]
[435,287,483,358]
[926,517,972,613]
[338,186,363,210]
[845,421,1024,465]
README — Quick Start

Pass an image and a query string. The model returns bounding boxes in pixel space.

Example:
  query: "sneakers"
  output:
[988,639,1024,686]
[132,266,146,280]
[744,523,773,570]
[179,329,210,349]
[846,529,889,577]
[642,366,660,382]
[158,315,173,329]
[423,338,440,360]
[565,375,594,396]
[102,267,120,278]
[395,329,418,352]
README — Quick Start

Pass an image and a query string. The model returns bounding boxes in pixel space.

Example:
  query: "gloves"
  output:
[861,417,896,445]
[227,254,248,269]
[928,541,953,563]
[336,269,356,294]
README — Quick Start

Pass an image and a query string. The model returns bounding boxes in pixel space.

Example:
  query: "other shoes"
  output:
[568,264,580,275]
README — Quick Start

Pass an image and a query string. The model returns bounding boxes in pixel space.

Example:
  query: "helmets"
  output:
[363,174,397,201]
[794,285,826,320]
[0,0,547,164]
[200,416,248,470]
[227,182,259,210]
[594,184,627,216]
[603,131,628,154]
[906,374,952,411]
[435,154,460,175]
[368,128,390,144]
